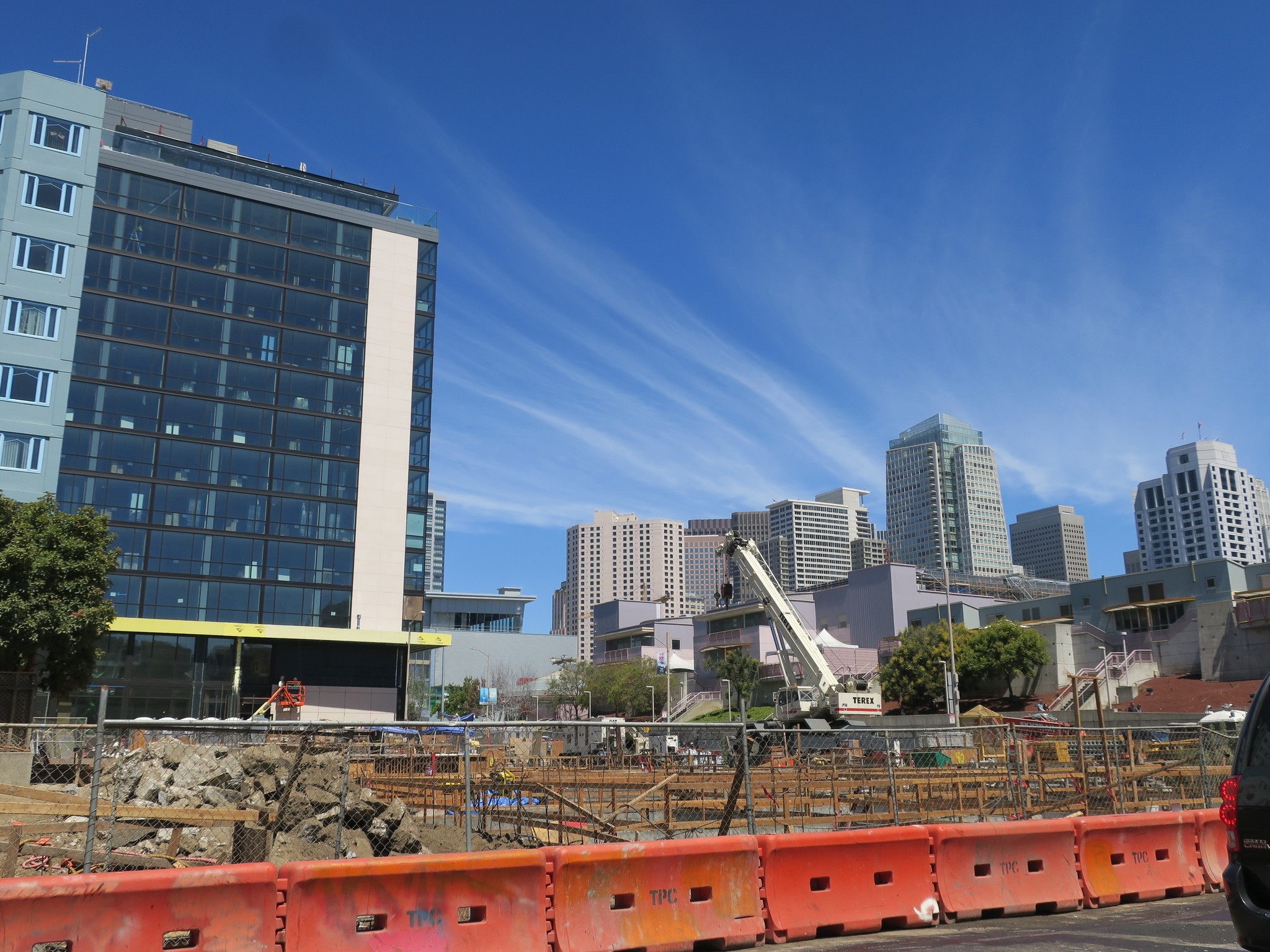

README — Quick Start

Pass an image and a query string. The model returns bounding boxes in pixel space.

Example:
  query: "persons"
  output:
[1037,700,1050,711]
[1135,704,1143,712]
[658,652,666,664]
[1128,701,1137,712]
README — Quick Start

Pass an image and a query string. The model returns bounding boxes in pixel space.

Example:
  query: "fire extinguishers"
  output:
[424,763,433,784]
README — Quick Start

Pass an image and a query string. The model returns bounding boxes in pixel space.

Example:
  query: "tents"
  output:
[364,726,426,756]
[765,628,859,664]
[665,652,694,674]
[417,727,475,754]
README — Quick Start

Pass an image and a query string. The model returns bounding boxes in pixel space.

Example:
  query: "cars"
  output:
[1217,666,1270,950]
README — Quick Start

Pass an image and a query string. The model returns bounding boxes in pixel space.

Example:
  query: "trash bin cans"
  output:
[905,752,952,767]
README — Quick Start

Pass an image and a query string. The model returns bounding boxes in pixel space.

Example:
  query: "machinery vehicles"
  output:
[712,526,973,765]
[1001,712,1088,761]
[246,681,306,720]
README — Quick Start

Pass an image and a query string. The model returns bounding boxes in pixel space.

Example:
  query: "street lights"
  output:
[644,632,672,733]
[917,569,962,727]
[934,660,949,716]
[404,610,426,723]
[1097,646,1112,710]
[721,679,731,721]
[646,685,655,723]
[532,696,538,730]
[680,681,683,706]
[584,691,591,719]
[1120,631,1130,687]
[470,648,489,722]
